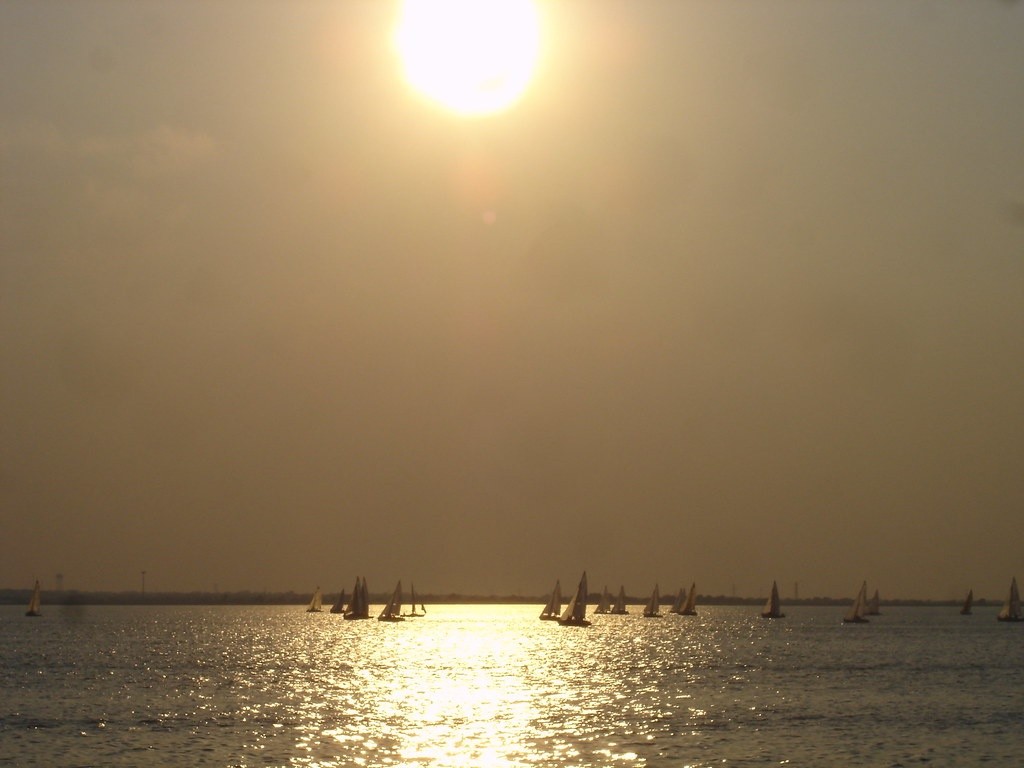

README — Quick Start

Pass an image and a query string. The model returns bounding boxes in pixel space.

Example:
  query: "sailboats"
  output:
[670,580,700,617]
[307,586,324,614]
[762,580,786,619]
[997,577,1024,621]
[330,587,349,615]
[594,585,611,616]
[23,579,44,617]
[843,580,882,625]
[642,582,665,618]
[344,577,426,623]
[539,568,591,627]
[610,586,630,615]
[960,588,975,617]
